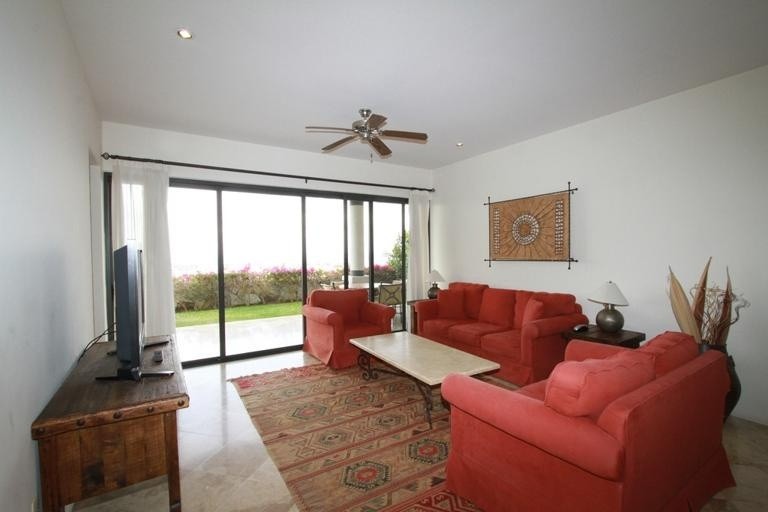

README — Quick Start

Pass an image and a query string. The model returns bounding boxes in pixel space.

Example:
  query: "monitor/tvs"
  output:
[96,245,175,380]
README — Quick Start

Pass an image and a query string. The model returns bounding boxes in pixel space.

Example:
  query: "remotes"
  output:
[154,350,162,362]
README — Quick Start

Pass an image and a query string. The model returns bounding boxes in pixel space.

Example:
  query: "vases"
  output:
[691,338,742,426]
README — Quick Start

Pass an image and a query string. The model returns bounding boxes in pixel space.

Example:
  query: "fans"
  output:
[306,109,428,158]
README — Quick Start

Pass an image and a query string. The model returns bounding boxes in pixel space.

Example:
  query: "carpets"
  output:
[227,361,520,511]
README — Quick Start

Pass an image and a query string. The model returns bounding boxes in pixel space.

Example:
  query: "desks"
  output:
[408,300,421,333]
[568,325,647,350]
[33,332,190,511]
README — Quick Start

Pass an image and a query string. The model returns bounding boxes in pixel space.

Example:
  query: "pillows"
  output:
[434,282,574,335]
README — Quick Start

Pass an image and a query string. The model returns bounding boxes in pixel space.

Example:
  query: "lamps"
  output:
[424,268,447,299]
[589,280,631,334]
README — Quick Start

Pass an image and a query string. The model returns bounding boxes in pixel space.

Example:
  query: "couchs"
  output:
[413,283,588,387]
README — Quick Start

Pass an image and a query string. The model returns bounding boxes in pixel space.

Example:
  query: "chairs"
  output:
[317,282,340,290]
[439,331,736,512]
[301,288,395,370]
[379,283,402,322]
